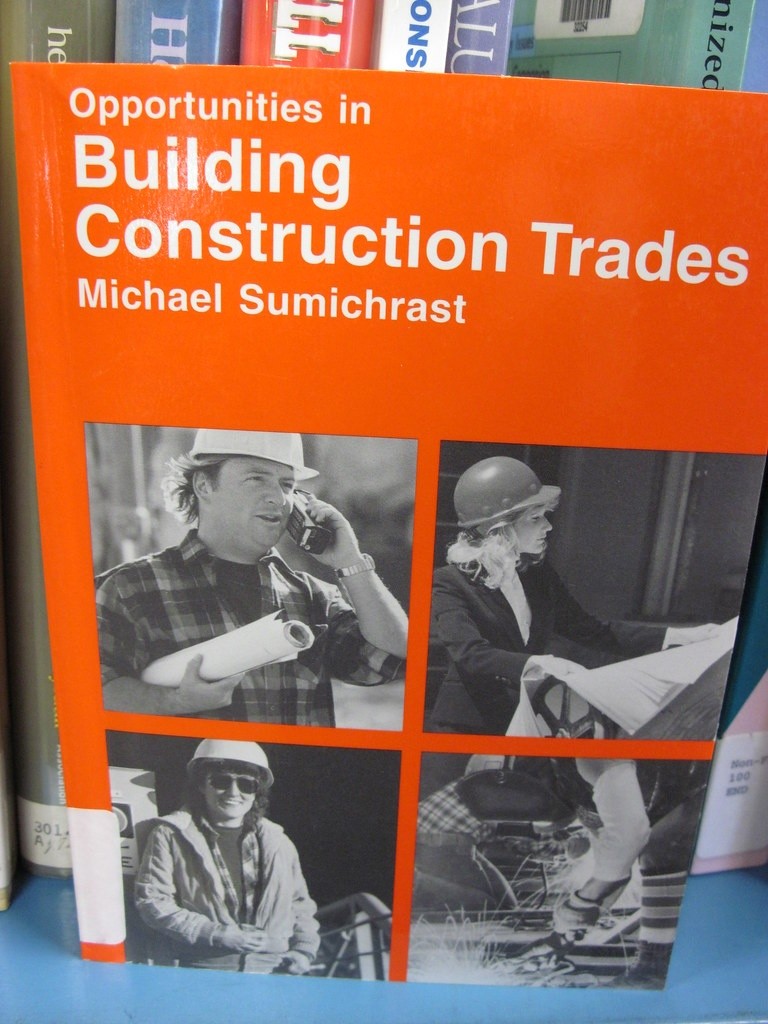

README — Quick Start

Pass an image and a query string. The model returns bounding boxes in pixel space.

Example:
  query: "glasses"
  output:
[202,773,262,794]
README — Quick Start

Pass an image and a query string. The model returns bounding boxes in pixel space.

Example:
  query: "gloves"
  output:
[554,889,603,941]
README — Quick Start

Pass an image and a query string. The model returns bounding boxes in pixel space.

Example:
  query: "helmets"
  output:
[190,428,320,481]
[186,740,274,790]
[454,456,561,528]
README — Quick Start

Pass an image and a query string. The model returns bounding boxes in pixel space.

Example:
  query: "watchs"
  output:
[335,554,375,578]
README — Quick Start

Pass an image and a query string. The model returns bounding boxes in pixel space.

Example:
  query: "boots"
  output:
[593,938,675,989]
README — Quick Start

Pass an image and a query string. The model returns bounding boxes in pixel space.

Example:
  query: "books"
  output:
[2,0,762,994]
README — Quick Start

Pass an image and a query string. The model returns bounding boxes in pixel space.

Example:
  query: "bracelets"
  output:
[570,890,603,907]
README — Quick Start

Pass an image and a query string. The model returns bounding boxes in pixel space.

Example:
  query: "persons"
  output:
[512,756,711,983]
[133,738,321,975]
[423,456,719,739]
[93,429,409,728]
